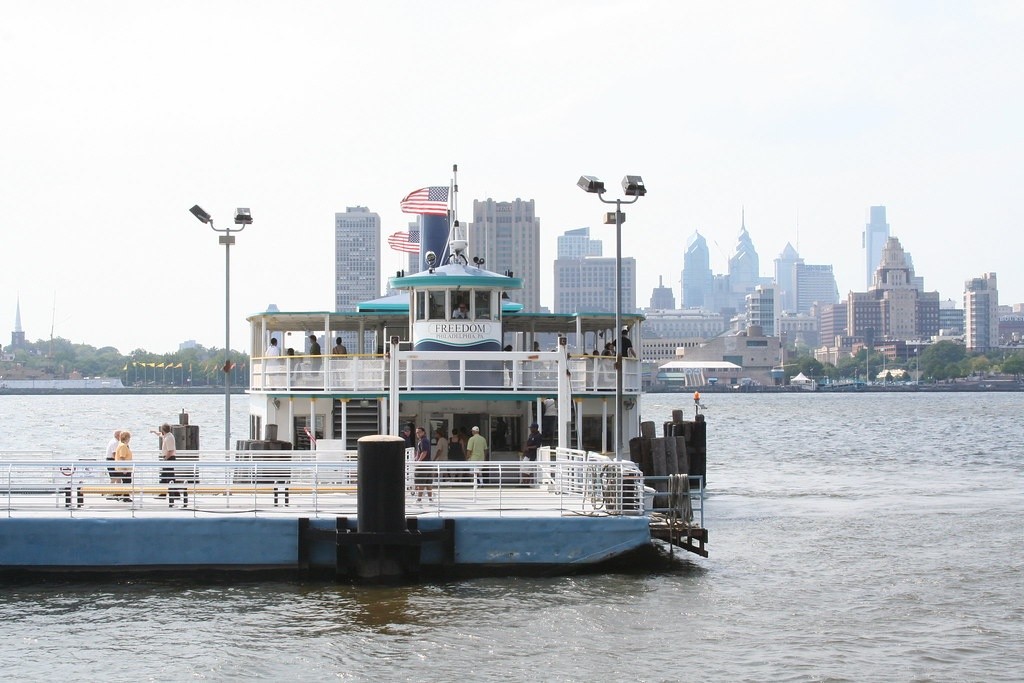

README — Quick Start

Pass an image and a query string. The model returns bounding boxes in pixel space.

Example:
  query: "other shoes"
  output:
[123,498,132,502]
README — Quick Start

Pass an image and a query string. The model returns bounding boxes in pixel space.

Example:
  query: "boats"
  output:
[242,160,644,492]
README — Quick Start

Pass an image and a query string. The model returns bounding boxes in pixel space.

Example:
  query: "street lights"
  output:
[190,203,254,491]
[573,173,648,518]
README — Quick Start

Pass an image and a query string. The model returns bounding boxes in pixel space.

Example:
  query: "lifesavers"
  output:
[59,466,76,476]
[304,427,317,444]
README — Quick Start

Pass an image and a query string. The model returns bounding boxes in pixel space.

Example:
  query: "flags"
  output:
[388,231,420,254]
[400,184,449,216]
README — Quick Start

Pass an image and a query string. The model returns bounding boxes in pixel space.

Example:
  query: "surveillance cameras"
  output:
[425,251,436,262]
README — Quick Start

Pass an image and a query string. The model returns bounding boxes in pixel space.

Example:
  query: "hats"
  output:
[470,426,479,432]
[528,423,538,428]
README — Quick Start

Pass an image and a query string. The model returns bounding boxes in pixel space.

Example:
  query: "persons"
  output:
[542,398,558,446]
[413,426,433,503]
[611,330,636,358]
[150,424,181,499]
[519,422,542,487]
[332,337,347,358]
[400,425,417,490]
[466,426,490,489]
[285,348,295,378]
[502,345,513,352]
[106,430,123,500]
[452,302,468,319]
[593,342,613,356]
[114,432,133,502]
[432,428,454,488]
[494,416,508,450]
[529,341,540,352]
[309,335,322,377]
[265,337,285,391]
[447,424,471,482]
